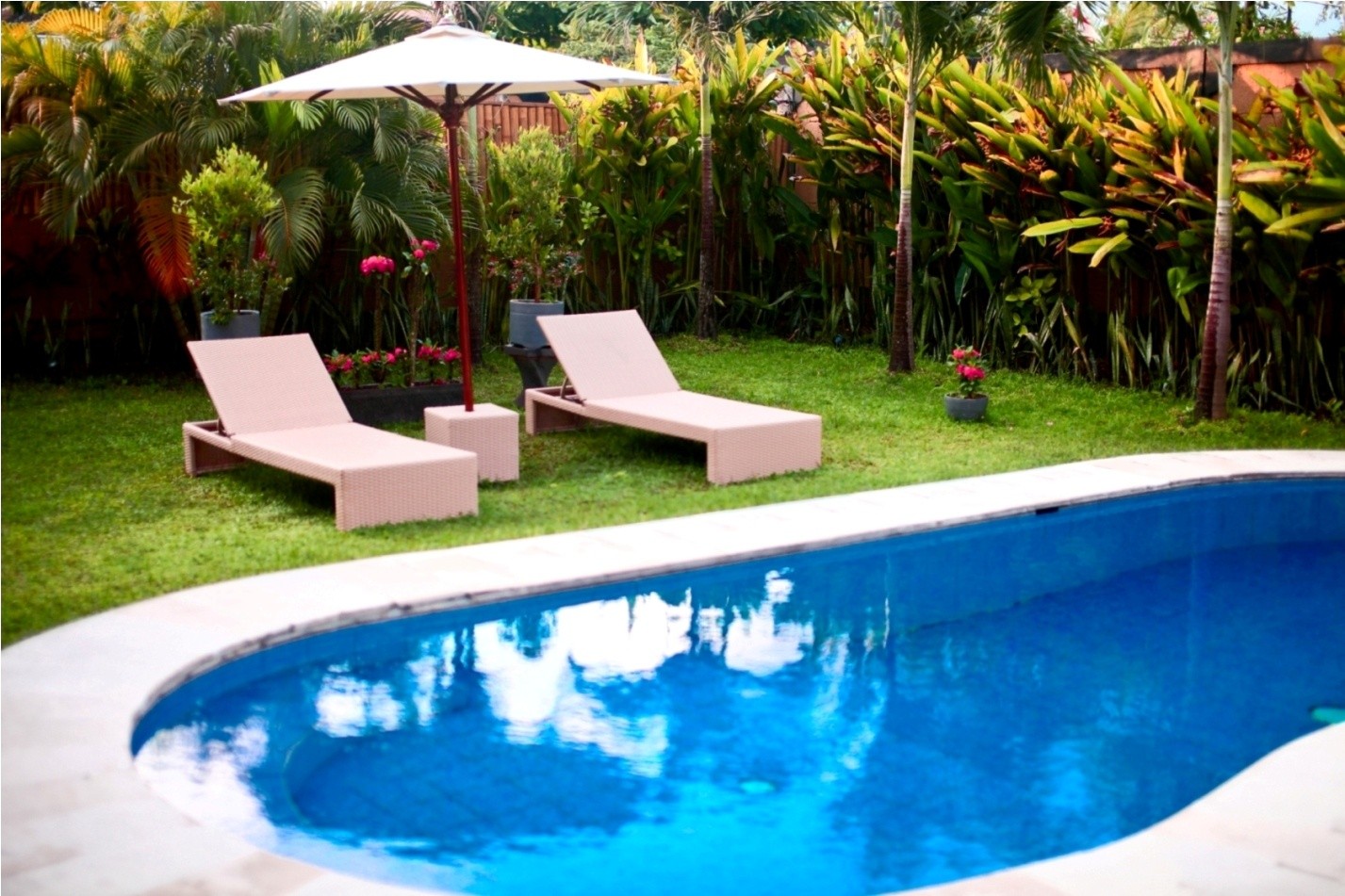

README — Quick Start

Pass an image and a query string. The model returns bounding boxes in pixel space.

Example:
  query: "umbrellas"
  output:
[216,9,675,411]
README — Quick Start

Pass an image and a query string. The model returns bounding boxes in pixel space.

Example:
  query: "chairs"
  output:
[525,306,823,485]
[181,333,480,533]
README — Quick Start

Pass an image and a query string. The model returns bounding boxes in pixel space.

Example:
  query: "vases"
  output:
[340,380,463,426]
[199,310,259,339]
[945,392,988,422]
[511,300,565,351]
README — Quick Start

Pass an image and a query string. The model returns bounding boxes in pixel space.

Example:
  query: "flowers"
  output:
[483,130,598,301]
[170,144,294,324]
[946,343,985,397]
[324,238,461,387]
